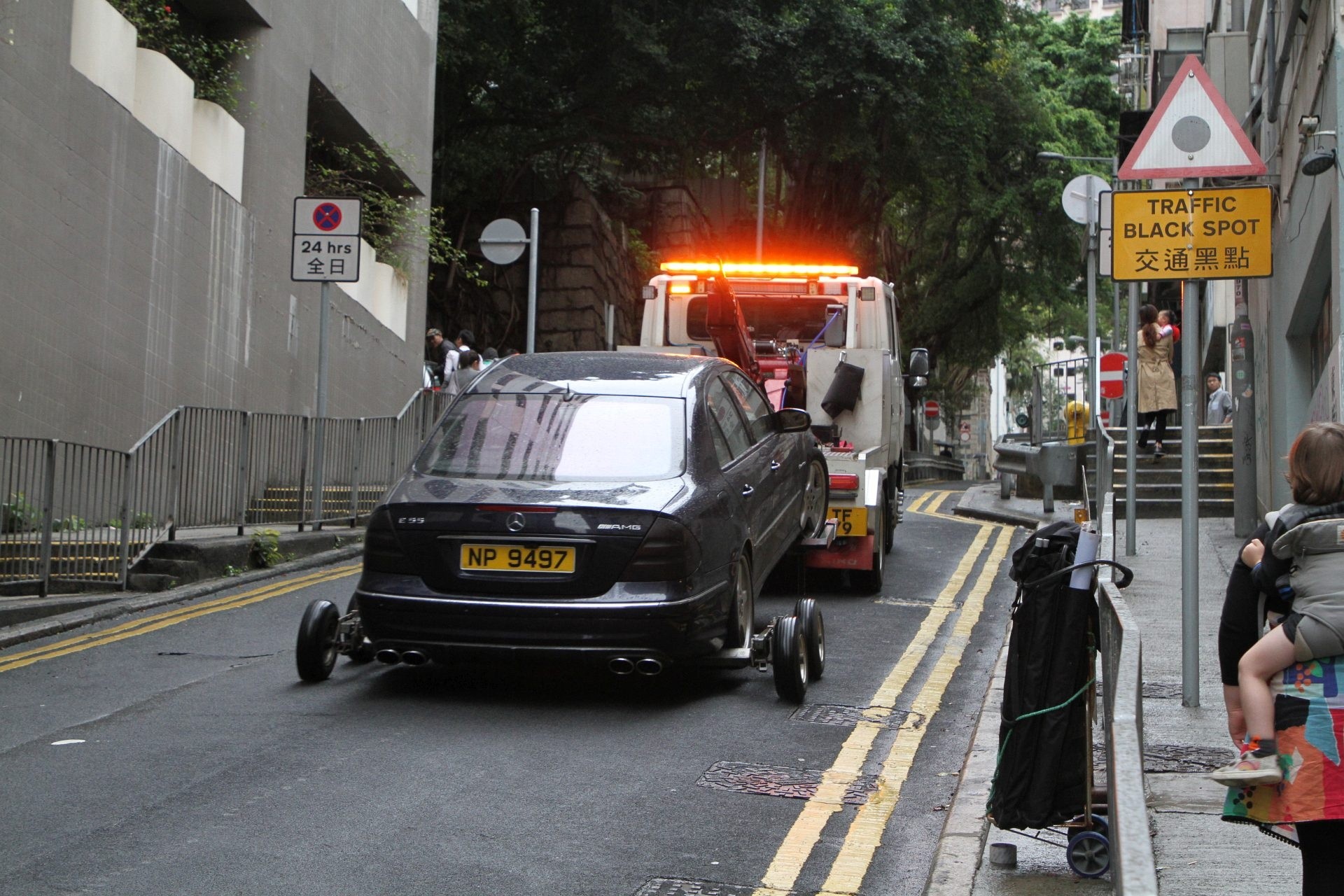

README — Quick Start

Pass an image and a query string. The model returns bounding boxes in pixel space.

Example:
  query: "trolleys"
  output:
[990,514,1115,876]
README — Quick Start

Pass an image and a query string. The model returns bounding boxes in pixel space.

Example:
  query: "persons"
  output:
[1212,536,1344,788]
[1135,304,1178,455]
[442,350,481,436]
[1180,424,1344,896]
[481,347,498,369]
[425,328,458,385]
[500,348,520,356]
[1157,310,1177,364]
[1203,372,1233,425]
[443,330,483,389]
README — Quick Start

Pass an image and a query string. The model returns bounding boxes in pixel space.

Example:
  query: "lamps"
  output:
[1297,115,1336,137]
[1301,145,1338,176]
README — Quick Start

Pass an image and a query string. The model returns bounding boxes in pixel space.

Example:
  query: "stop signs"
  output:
[925,400,939,417]
[1100,353,1129,399]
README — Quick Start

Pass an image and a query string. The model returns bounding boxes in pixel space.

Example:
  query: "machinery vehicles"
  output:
[638,260,929,591]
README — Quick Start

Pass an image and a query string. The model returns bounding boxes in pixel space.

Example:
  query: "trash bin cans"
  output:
[1064,402,1089,446]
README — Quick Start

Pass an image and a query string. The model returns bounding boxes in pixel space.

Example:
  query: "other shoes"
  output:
[1154,447,1166,456]
[1211,753,1282,788]
[1137,425,1150,447]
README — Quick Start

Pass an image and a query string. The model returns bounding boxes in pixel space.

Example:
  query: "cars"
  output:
[355,351,829,677]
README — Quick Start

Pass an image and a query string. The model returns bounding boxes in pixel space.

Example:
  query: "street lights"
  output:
[1038,148,1120,426]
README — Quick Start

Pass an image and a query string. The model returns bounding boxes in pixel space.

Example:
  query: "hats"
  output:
[425,328,442,337]
